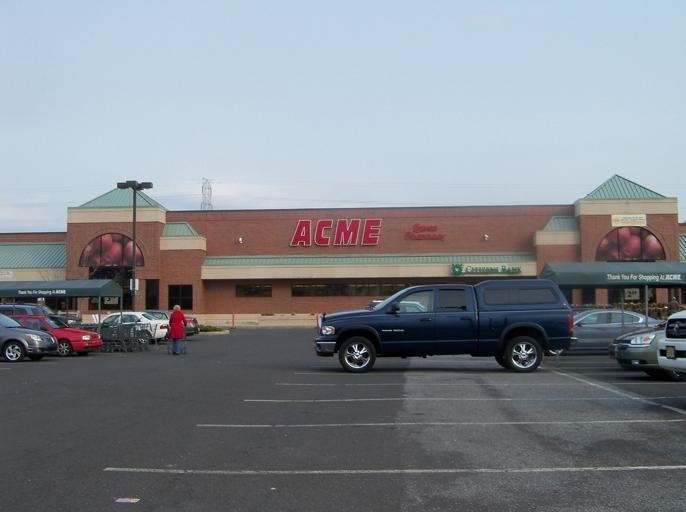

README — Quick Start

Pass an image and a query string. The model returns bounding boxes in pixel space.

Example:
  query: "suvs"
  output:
[314,279,573,373]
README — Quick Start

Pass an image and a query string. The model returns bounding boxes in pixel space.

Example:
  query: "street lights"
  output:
[117,181,153,311]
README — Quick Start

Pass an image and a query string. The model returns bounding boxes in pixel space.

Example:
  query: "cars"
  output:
[144,310,199,340]
[609,309,686,376]
[0,303,104,362]
[104,311,169,344]
[371,300,427,313]
[567,308,664,355]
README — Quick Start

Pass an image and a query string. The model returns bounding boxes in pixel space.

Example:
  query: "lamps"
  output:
[484,233,489,240]
[238,237,243,243]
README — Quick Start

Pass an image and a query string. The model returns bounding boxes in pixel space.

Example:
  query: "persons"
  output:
[170,305,187,355]
[669,296,680,315]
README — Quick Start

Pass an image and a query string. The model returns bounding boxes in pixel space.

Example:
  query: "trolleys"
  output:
[167,326,187,355]
[102,323,160,353]
